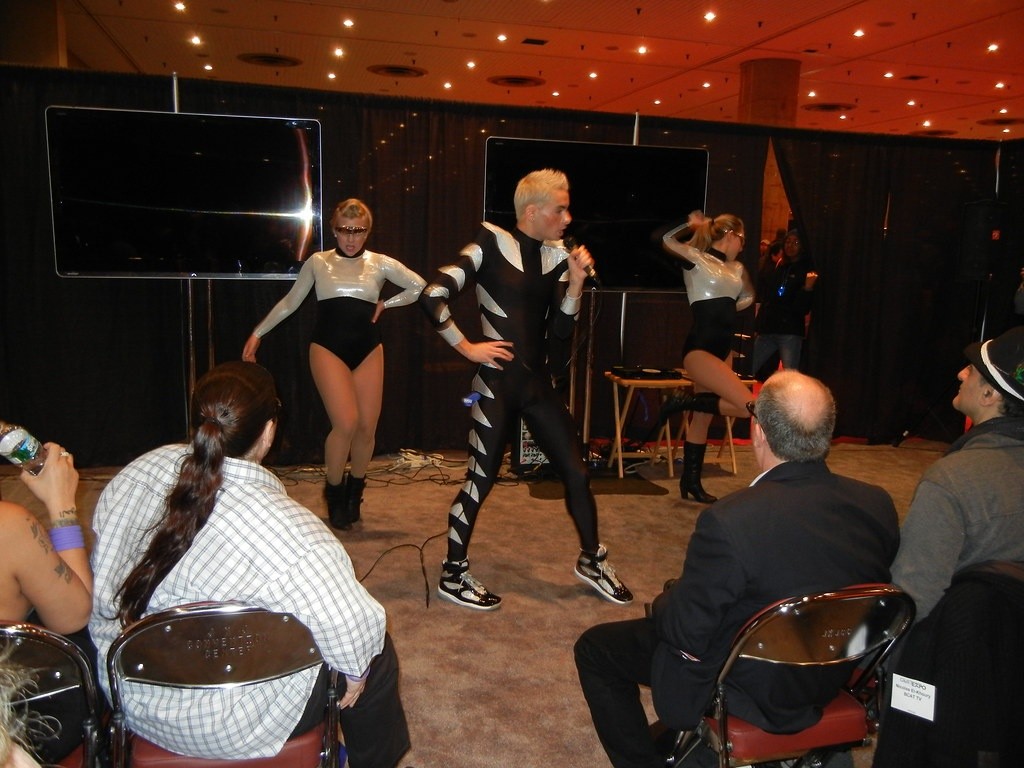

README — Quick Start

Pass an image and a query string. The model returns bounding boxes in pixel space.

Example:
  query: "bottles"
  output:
[0,420,48,477]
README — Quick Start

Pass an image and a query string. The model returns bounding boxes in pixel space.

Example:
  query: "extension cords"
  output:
[398,449,440,469]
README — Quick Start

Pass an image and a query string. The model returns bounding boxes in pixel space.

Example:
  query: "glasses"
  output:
[784,239,801,247]
[746,401,759,419]
[724,229,745,245]
[335,225,368,235]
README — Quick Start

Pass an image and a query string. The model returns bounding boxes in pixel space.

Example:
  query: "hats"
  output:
[965,326,1024,404]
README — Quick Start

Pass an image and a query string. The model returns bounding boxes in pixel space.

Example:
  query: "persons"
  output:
[857,325,1024,682]
[0,441,93,634]
[88,361,411,768]
[574,369,901,768]
[421,168,633,611]
[658,208,757,503]
[753,224,818,383]
[243,196,428,529]
[0,637,66,768]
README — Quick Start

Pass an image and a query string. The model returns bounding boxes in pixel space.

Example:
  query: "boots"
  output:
[325,475,353,530]
[680,440,717,503]
[658,389,720,426]
[346,471,366,523]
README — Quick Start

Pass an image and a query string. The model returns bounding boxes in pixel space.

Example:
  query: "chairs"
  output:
[0,621,108,768]
[666,584,916,768]
[107,602,342,768]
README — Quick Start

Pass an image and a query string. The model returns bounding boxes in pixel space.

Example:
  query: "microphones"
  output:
[564,234,600,285]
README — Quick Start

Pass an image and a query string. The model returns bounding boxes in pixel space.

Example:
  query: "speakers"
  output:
[510,377,569,474]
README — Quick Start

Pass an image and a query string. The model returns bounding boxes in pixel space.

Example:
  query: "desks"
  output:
[604,371,756,478]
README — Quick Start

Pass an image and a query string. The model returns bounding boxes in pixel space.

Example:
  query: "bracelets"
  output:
[50,524,85,552]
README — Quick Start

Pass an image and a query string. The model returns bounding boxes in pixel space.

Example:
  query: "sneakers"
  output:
[437,555,502,610]
[574,544,634,604]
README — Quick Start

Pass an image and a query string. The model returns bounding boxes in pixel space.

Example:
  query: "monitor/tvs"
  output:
[45,105,323,280]
[484,135,710,293]
